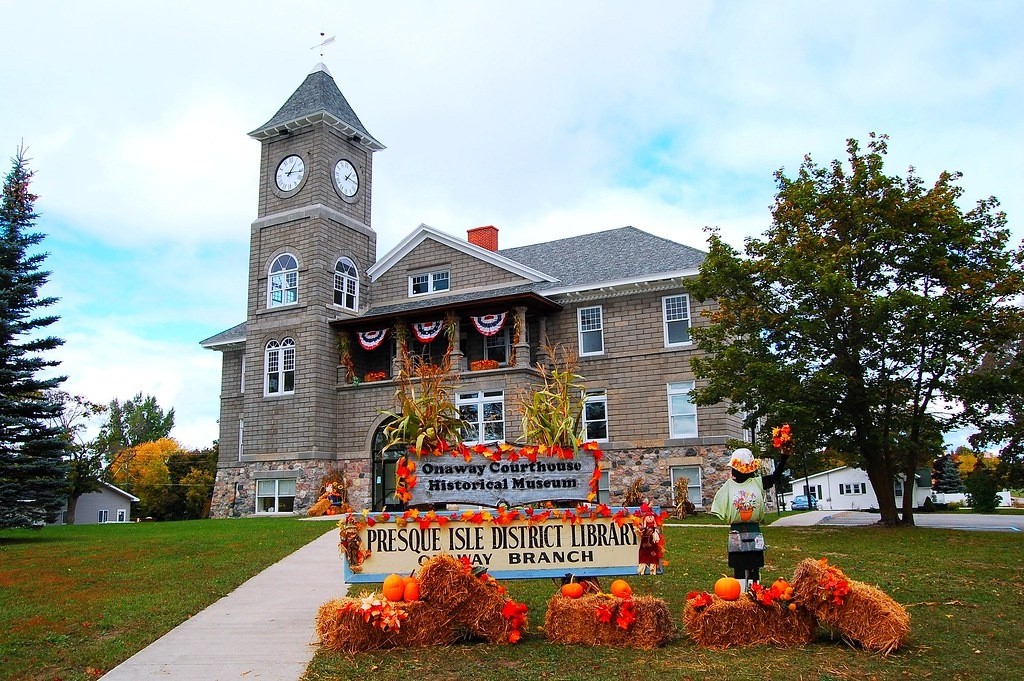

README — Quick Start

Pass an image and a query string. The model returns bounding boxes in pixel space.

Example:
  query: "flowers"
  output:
[730,459,762,474]
[470,360,500,370]
[321,472,345,493]
[338,506,670,571]
[389,443,604,501]
[364,371,387,382]
[770,423,794,451]
[357,591,408,634]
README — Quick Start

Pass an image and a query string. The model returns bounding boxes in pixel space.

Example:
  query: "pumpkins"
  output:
[714,574,741,600]
[610,580,632,598]
[326,508,335,515]
[562,573,583,598]
[772,577,789,594]
[382,568,421,603]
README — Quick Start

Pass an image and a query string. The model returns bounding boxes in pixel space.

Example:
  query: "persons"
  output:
[710,447,791,582]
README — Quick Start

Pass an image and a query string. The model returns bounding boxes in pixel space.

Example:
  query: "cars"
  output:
[157,511,175,521]
[790,495,818,511]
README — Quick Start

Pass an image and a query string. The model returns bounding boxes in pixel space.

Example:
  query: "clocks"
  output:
[270,150,310,198]
[331,156,364,204]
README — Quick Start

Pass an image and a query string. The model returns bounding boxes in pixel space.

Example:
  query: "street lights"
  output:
[745,399,760,446]
